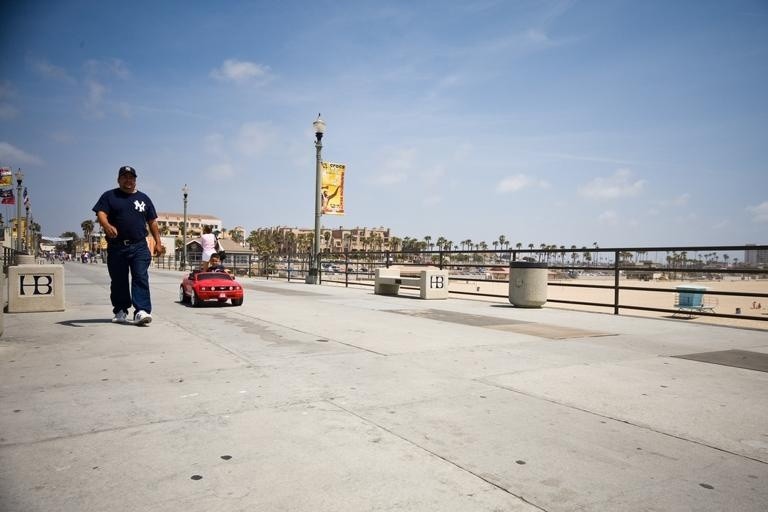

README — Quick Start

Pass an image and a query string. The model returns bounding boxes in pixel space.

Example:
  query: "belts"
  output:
[113,239,137,246]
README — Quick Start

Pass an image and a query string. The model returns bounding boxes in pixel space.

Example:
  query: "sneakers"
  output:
[112,310,129,323]
[134,310,152,326]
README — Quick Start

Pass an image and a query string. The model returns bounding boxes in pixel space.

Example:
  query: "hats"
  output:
[119,166,138,178]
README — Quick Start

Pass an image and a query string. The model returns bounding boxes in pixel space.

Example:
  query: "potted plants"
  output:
[305,268,319,284]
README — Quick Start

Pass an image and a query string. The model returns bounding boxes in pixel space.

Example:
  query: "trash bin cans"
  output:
[14,254,36,265]
[508,261,548,308]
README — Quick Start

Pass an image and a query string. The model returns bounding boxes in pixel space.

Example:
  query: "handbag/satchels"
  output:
[215,240,226,260]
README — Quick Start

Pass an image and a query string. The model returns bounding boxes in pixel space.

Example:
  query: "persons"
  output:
[206,252,227,272]
[201,227,218,271]
[91,165,162,324]
[37,248,90,266]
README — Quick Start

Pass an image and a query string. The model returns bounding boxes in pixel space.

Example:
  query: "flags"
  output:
[22,187,29,206]
[0,167,16,206]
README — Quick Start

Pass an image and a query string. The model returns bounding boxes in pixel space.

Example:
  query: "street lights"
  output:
[302,112,325,284]
[24,204,29,254]
[180,181,189,268]
[14,167,26,254]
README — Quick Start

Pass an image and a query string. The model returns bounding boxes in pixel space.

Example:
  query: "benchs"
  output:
[374,267,448,300]
[8,263,65,313]
